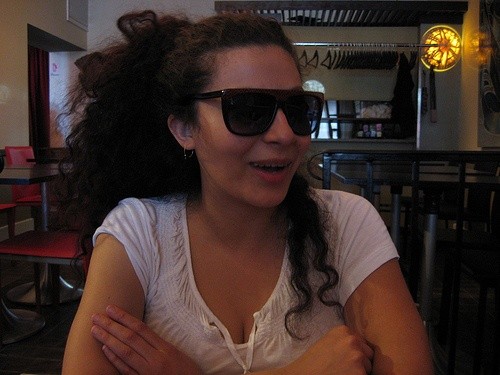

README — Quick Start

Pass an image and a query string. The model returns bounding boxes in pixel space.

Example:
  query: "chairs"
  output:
[0,145,84,304]
[322,151,500,375]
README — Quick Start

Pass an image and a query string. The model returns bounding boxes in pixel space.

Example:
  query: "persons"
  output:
[50,9,434,375]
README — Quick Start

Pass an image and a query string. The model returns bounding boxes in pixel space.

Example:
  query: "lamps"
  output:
[420,24,462,72]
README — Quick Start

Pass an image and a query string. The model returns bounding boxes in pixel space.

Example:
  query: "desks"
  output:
[0,163,85,304]
[318,162,500,321]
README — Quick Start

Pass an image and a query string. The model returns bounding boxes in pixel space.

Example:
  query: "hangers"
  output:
[298,40,417,70]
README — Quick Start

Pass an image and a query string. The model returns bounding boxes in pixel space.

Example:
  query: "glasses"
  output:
[190,88,324,136]
